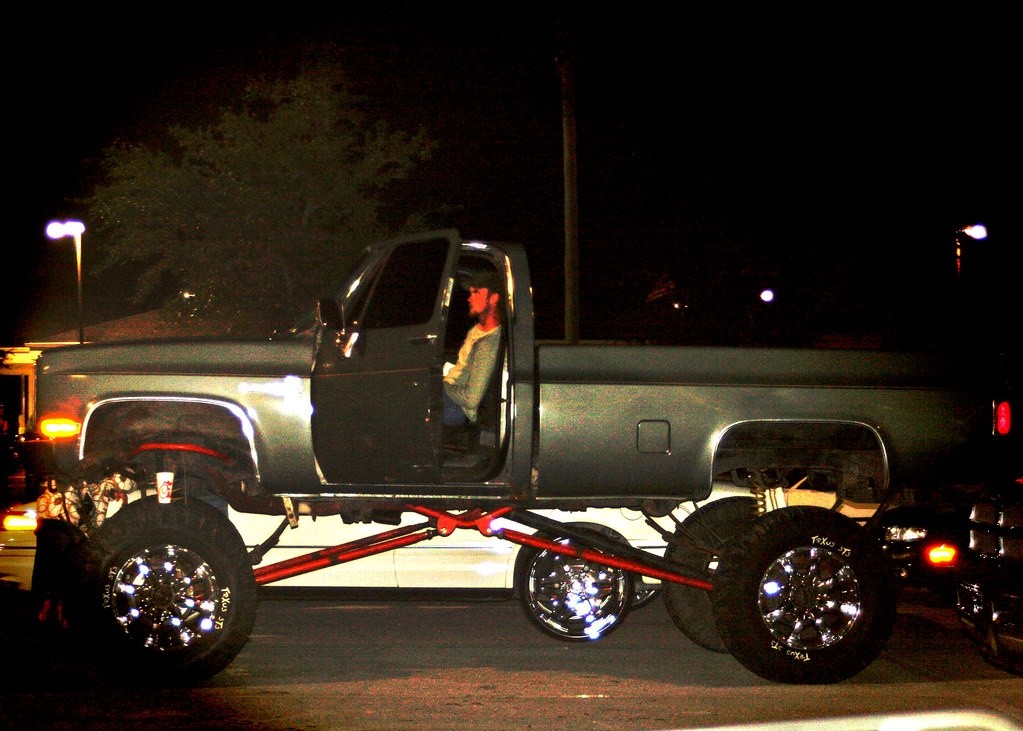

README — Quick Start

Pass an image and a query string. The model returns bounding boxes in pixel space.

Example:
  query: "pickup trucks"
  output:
[24,231,985,697]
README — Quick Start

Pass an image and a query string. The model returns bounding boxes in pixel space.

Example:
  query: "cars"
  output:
[865,492,1023,679]
[0,482,767,642]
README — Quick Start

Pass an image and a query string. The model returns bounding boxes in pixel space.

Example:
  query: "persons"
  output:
[442,269,514,432]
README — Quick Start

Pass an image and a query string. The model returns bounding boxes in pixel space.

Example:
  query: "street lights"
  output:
[45,222,89,348]
[950,225,989,369]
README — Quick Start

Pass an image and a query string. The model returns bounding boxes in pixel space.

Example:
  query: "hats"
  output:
[461,265,500,286]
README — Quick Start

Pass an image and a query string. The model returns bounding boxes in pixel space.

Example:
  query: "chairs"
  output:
[443,355,500,454]
